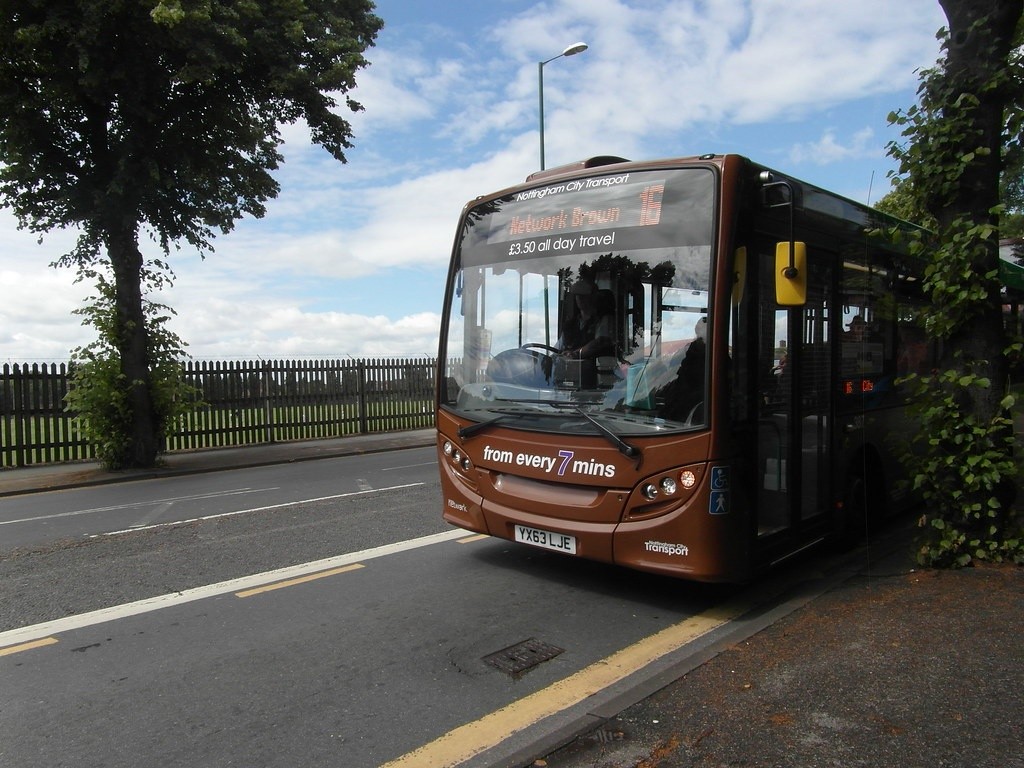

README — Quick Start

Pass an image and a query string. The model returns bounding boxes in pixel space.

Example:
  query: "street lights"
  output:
[538,41,588,173]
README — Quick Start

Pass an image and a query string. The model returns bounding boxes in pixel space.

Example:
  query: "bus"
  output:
[432,149,940,595]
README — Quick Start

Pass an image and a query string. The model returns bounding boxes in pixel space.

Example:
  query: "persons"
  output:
[645,316,735,425]
[550,279,613,383]
[846,314,868,336]
